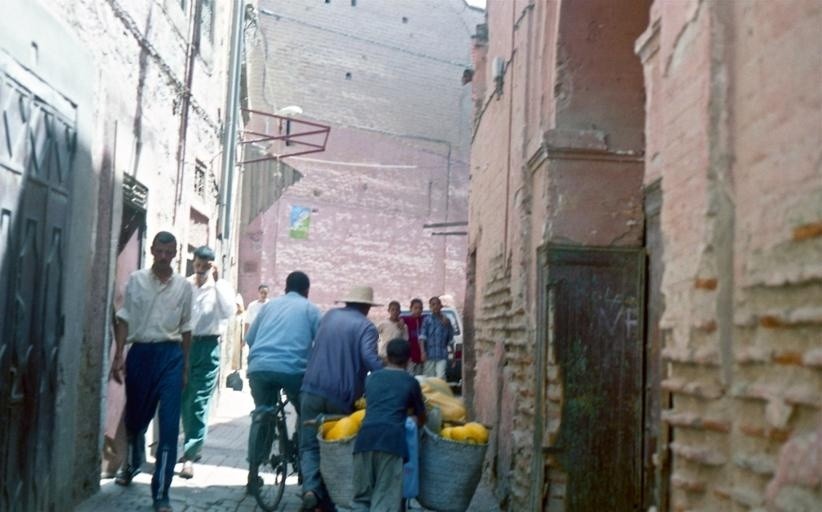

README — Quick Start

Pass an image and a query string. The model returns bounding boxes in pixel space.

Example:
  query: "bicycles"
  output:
[248,383,302,512]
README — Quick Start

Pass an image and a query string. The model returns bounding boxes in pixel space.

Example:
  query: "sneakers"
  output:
[178,455,202,466]
[155,501,169,511]
[299,490,320,512]
[247,475,266,493]
[179,465,194,479]
[115,475,131,486]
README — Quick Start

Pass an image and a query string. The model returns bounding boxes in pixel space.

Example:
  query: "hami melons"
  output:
[320,410,365,441]
[441,421,490,446]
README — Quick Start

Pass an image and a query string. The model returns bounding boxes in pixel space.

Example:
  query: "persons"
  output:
[242,285,274,353]
[372,300,409,364]
[247,272,322,493]
[398,300,425,375]
[351,339,428,511]
[178,246,236,479]
[299,283,386,511]
[107,232,195,512]
[419,297,454,380]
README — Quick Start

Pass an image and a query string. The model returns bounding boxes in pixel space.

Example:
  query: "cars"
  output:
[395,307,465,377]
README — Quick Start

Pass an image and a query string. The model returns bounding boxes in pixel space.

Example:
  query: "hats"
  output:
[334,286,384,306]
